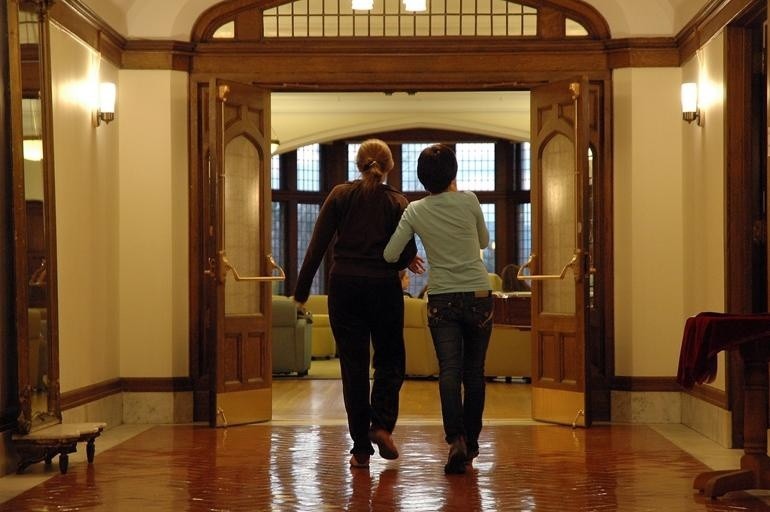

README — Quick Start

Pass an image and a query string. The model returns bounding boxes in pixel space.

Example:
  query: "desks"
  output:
[684,315,770,501]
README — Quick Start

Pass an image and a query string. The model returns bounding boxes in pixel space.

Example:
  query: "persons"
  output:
[383,143,494,476]
[292,137,417,470]
[501,264,531,292]
[398,268,414,299]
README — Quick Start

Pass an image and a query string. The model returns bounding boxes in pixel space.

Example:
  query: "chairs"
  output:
[270,291,532,385]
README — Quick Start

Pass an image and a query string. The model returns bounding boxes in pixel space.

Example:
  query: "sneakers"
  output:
[350,454,369,467]
[445,435,478,473]
[370,428,398,460]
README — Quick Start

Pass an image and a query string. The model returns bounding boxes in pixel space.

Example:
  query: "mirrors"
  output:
[4,2,60,433]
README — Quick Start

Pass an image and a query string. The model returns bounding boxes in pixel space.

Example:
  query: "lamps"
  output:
[93,83,116,126]
[680,82,706,127]
